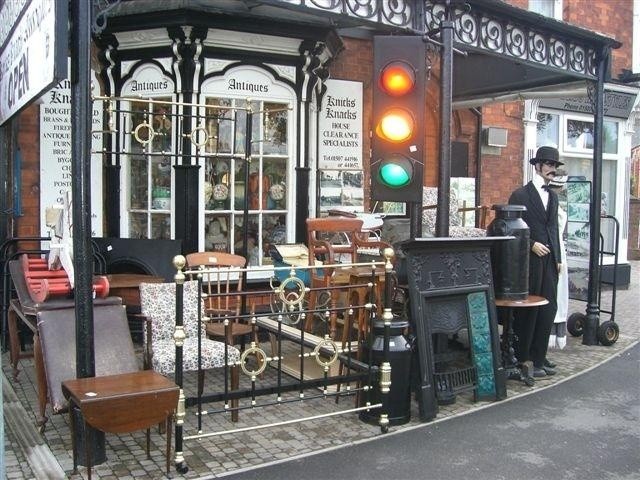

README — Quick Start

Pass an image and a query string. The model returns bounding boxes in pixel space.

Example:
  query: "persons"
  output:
[509,146,565,378]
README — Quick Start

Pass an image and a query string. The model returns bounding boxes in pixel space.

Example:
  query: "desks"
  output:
[8,299,48,434]
[494,294,550,387]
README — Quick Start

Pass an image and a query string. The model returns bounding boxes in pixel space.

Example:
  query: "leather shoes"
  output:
[534,365,557,377]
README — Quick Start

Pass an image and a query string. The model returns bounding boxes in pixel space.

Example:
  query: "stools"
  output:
[61,369,180,480]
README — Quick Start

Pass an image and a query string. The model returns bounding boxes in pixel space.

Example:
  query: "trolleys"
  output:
[567,214,620,346]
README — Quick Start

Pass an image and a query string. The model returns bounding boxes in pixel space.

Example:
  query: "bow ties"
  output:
[541,184,549,191]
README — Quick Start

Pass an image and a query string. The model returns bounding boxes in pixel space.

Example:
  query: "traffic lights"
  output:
[370,34,428,204]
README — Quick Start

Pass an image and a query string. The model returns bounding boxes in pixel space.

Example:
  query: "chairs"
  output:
[137,252,264,434]
[303,218,396,350]
[422,186,489,238]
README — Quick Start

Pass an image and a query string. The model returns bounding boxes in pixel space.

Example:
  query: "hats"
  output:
[530,146,564,166]
